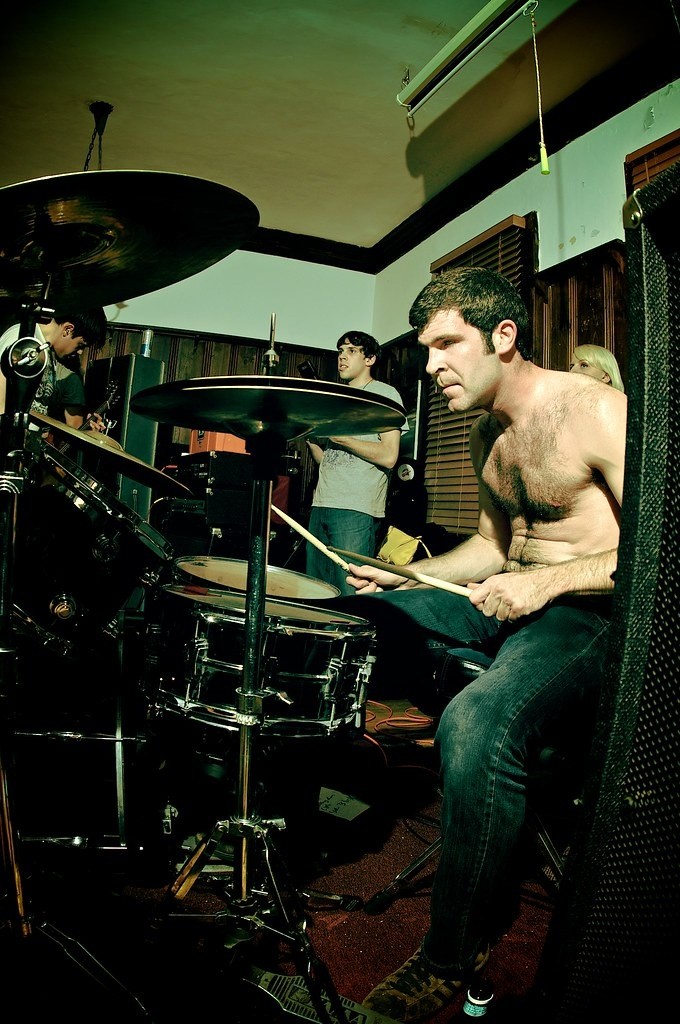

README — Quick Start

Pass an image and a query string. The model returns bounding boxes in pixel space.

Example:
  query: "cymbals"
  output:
[29,409,194,498]
[0,167,261,314]
[129,373,407,439]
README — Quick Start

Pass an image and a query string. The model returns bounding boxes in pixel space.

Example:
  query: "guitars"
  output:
[41,379,122,491]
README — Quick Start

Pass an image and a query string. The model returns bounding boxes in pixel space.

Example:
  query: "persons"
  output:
[47,359,106,476]
[0,297,107,415]
[301,331,404,675]
[272,267,626,1024]
[569,345,624,393]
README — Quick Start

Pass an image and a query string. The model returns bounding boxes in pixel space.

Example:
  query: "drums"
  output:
[0,429,174,647]
[0,729,145,848]
[132,582,378,740]
[170,554,342,609]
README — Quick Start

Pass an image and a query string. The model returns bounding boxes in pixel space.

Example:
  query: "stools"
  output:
[363,645,593,919]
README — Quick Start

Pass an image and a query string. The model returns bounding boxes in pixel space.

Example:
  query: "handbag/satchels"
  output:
[376,526,432,566]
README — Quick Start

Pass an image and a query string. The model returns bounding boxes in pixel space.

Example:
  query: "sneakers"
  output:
[361,942,490,1024]
[196,832,235,862]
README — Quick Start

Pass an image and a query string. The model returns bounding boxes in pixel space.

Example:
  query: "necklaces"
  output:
[363,379,374,389]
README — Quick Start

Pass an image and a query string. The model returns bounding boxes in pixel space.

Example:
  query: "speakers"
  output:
[523,162,680,1024]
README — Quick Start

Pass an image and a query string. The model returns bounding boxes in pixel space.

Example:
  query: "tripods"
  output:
[0,244,349,1024]
